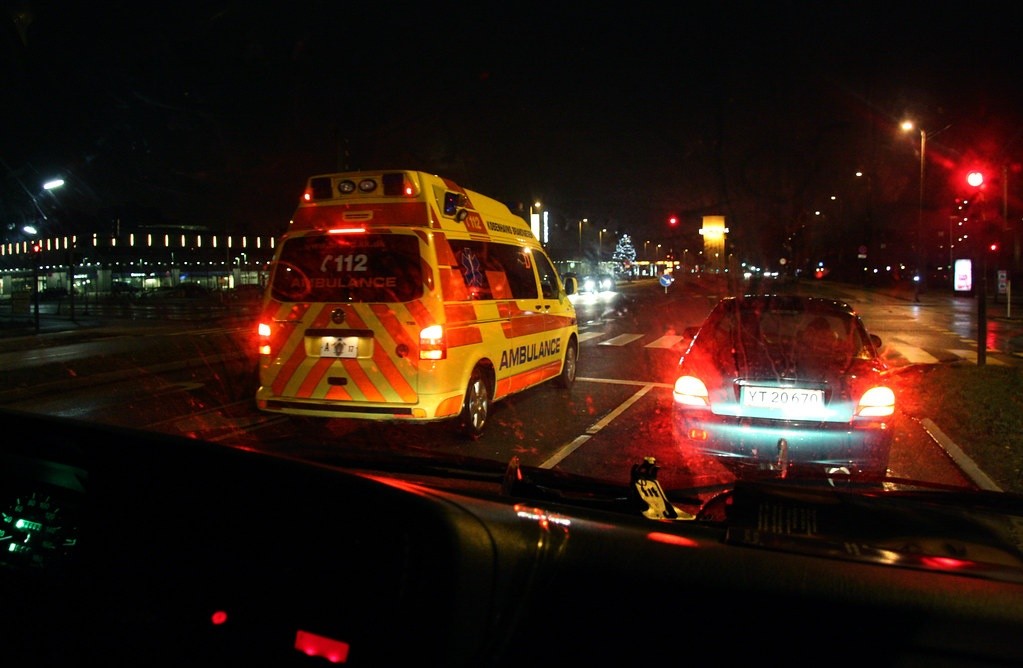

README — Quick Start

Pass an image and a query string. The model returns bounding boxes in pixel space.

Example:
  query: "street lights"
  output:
[656,244,662,260]
[682,249,689,274]
[529,201,541,230]
[901,119,928,294]
[598,228,607,274]
[578,218,588,260]
[643,241,650,260]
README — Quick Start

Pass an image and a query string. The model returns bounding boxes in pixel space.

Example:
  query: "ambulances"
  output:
[254,165,581,438]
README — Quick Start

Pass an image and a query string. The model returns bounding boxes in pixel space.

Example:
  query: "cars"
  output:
[560,270,629,319]
[669,291,899,484]
